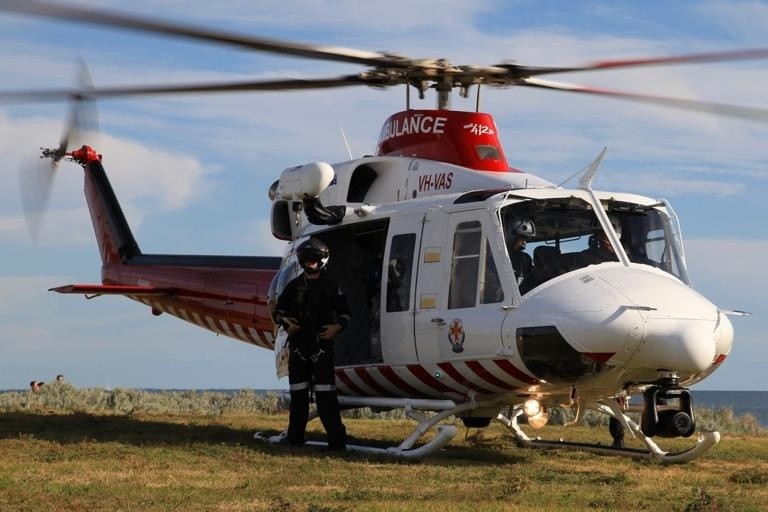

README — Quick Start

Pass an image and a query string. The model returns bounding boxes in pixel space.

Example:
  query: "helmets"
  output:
[591,215,622,241]
[297,239,330,274]
[504,216,537,243]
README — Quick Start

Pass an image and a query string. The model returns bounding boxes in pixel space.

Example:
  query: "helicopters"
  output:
[0,0,768,462]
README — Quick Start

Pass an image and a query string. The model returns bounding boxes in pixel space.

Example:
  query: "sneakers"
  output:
[322,445,345,452]
[280,437,304,447]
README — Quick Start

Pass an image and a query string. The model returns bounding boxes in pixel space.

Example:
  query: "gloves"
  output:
[279,317,300,335]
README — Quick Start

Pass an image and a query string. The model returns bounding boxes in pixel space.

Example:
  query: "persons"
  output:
[589,214,632,267]
[29,375,64,392]
[483,214,536,303]
[271,239,352,452]
